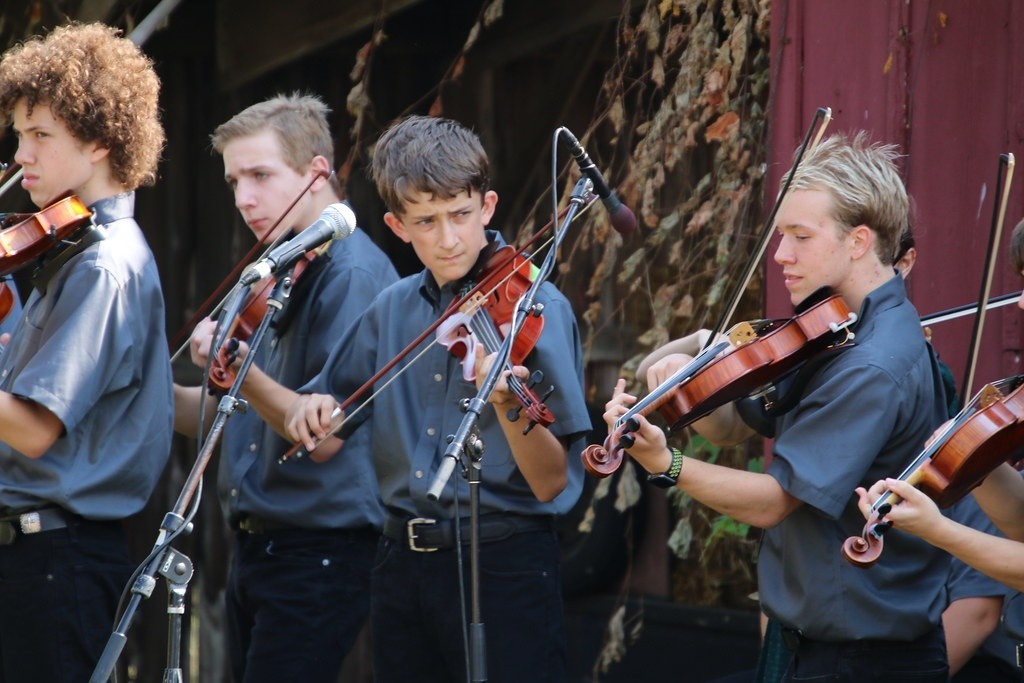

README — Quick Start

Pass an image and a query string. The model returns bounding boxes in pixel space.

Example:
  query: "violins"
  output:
[206,249,316,398]
[580,283,858,479]
[0,194,94,277]
[840,373,1024,570]
[434,240,555,436]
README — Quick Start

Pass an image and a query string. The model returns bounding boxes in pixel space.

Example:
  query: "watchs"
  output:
[646,447,682,489]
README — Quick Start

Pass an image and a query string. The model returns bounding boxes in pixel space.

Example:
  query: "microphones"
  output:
[560,126,638,234]
[237,202,357,287]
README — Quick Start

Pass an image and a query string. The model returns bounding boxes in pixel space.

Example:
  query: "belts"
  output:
[237,517,298,535]
[780,626,816,654]
[1,519,123,546]
[381,512,552,552]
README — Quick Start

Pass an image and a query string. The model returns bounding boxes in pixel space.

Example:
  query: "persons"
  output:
[604,131,950,683]
[287,116,593,683]
[759,219,1024,683]
[174,93,400,683]
[0,22,176,683]
